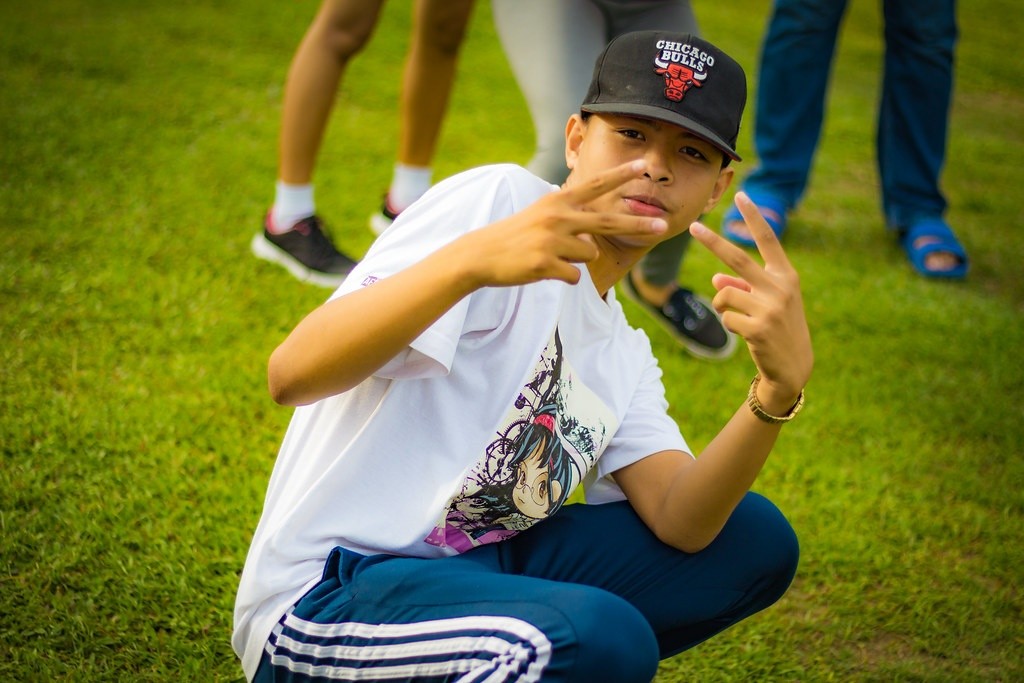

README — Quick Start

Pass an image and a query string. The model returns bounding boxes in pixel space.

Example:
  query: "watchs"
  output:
[748,372,805,424]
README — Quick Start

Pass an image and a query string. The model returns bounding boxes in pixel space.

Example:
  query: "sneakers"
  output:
[251,207,357,289]
[619,269,735,359]
[369,189,400,233]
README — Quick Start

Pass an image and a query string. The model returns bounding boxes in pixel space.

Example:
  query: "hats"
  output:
[581,28,747,162]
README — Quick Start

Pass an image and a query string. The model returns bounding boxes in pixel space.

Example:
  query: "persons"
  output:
[493,0,735,361]
[252,0,476,288]
[231,31,816,683]
[724,0,970,280]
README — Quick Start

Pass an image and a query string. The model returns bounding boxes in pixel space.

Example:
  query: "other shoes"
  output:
[898,212,968,277]
[724,185,790,246]
